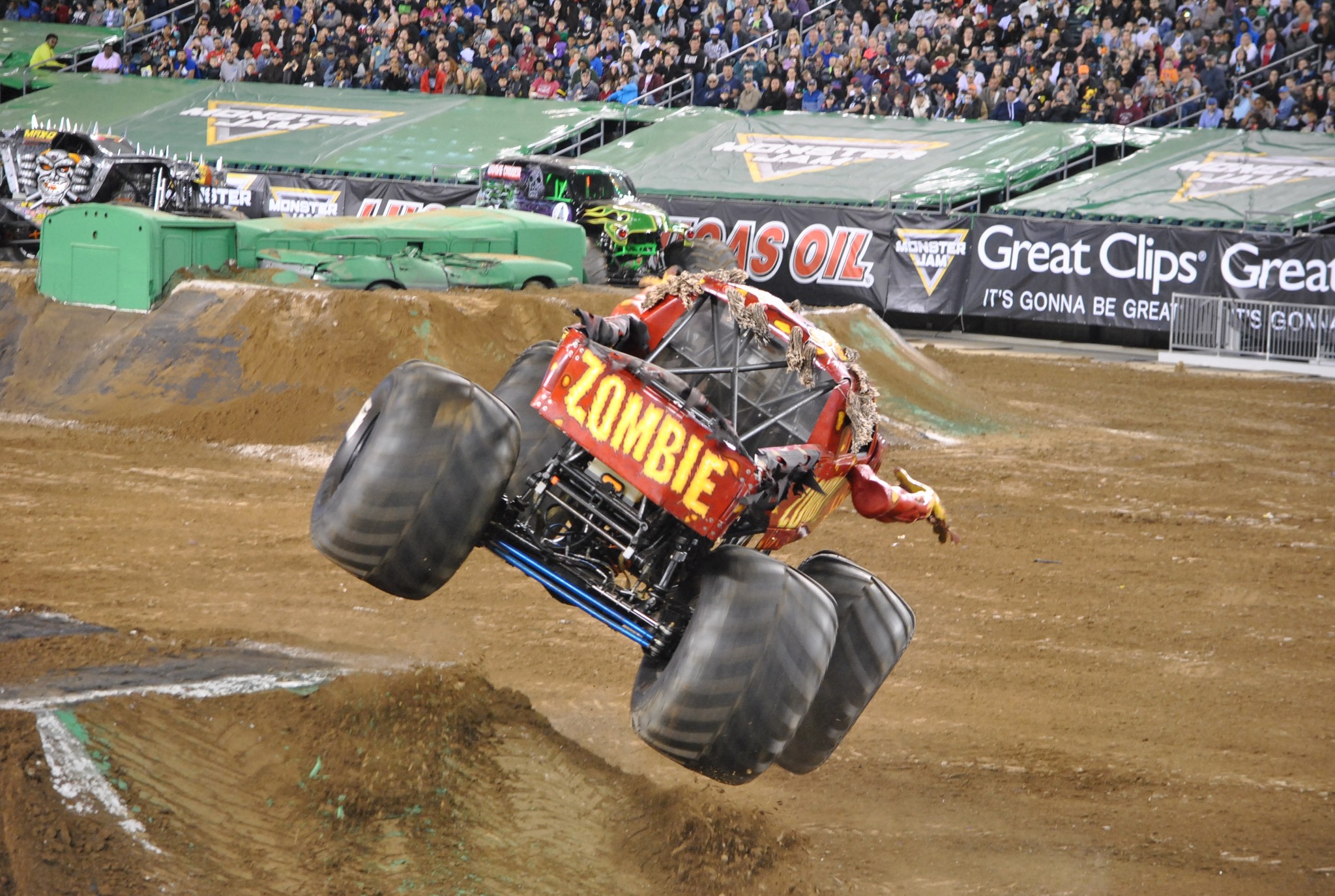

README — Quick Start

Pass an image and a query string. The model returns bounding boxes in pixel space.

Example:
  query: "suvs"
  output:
[0,126,177,261]
[478,155,679,285]
[312,238,962,781]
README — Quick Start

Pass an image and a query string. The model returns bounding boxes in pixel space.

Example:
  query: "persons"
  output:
[0,0,1335,132]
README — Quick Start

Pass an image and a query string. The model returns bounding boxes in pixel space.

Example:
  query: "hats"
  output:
[153,1,1335,140]
